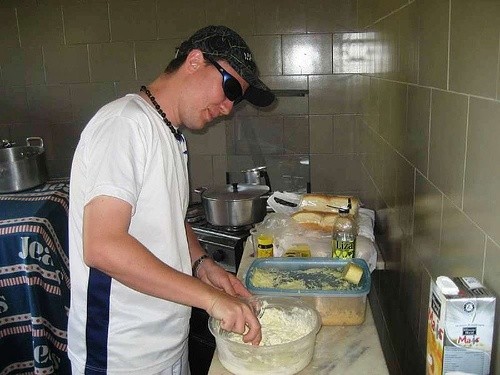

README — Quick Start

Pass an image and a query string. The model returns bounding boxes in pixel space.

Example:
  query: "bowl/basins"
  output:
[208,295,322,375]
[245,256,372,325]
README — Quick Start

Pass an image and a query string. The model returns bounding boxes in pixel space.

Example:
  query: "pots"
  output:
[0,137,47,193]
[194,183,274,227]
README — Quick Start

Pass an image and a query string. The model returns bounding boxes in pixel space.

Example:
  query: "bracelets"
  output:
[192,255,212,278]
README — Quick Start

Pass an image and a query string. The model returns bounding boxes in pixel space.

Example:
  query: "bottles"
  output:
[257,236,272,258]
[331,207,357,258]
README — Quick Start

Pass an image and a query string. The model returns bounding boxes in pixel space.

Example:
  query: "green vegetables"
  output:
[274,268,358,290]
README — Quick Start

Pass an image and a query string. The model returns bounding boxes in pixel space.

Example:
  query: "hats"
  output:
[189,26,274,108]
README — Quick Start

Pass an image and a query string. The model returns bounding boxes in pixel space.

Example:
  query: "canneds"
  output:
[258,235,273,258]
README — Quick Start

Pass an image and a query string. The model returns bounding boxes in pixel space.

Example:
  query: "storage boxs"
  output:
[245,257,372,325]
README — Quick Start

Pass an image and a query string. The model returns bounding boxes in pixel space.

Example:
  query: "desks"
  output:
[0,176,71,375]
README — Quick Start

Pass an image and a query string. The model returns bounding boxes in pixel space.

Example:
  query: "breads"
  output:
[291,192,361,234]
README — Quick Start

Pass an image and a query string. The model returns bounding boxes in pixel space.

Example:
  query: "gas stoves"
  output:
[185,201,254,274]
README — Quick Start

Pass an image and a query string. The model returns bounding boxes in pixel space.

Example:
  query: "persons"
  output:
[66,26,274,375]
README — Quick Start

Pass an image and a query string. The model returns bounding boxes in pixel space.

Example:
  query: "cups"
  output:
[241,166,272,197]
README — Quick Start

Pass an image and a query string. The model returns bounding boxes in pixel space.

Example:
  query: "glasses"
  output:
[202,52,246,107]
